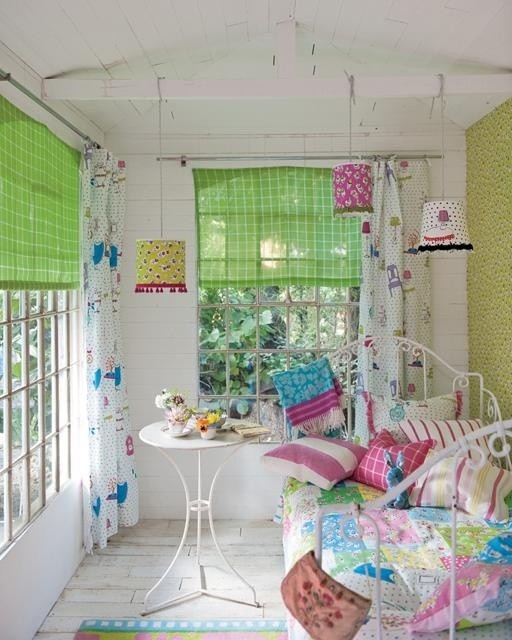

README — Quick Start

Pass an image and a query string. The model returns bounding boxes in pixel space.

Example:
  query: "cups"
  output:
[200,429,216,440]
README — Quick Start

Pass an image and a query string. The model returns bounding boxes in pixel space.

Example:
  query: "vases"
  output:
[168,421,186,435]
[200,425,217,439]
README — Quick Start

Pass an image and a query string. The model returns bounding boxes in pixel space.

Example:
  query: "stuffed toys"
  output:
[382,447,410,508]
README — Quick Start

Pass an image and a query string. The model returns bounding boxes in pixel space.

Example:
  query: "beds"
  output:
[269,336,512,640]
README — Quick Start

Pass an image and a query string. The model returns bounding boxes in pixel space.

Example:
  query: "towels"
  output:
[231,423,259,438]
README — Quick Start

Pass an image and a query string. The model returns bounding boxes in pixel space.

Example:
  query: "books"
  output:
[230,420,270,438]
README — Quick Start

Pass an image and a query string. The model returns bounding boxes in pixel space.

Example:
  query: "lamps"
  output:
[333,70,374,217]
[135,76,187,293]
[417,72,473,256]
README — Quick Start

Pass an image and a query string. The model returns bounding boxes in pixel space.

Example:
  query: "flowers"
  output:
[155,389,224,432]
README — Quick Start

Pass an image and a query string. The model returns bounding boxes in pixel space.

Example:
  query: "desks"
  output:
[139,416,260,616]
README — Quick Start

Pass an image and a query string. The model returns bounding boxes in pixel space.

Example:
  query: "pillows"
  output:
[352,428,437,499]
[408,532,512,634]
[355,390,464,452]
[393,419,495,463]
[408,456,512,524]
[262,434,367,491]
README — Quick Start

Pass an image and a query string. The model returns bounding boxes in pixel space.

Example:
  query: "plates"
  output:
[160,425,194,436]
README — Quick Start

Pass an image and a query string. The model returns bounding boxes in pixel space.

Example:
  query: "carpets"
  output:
[73,618,295,640]
